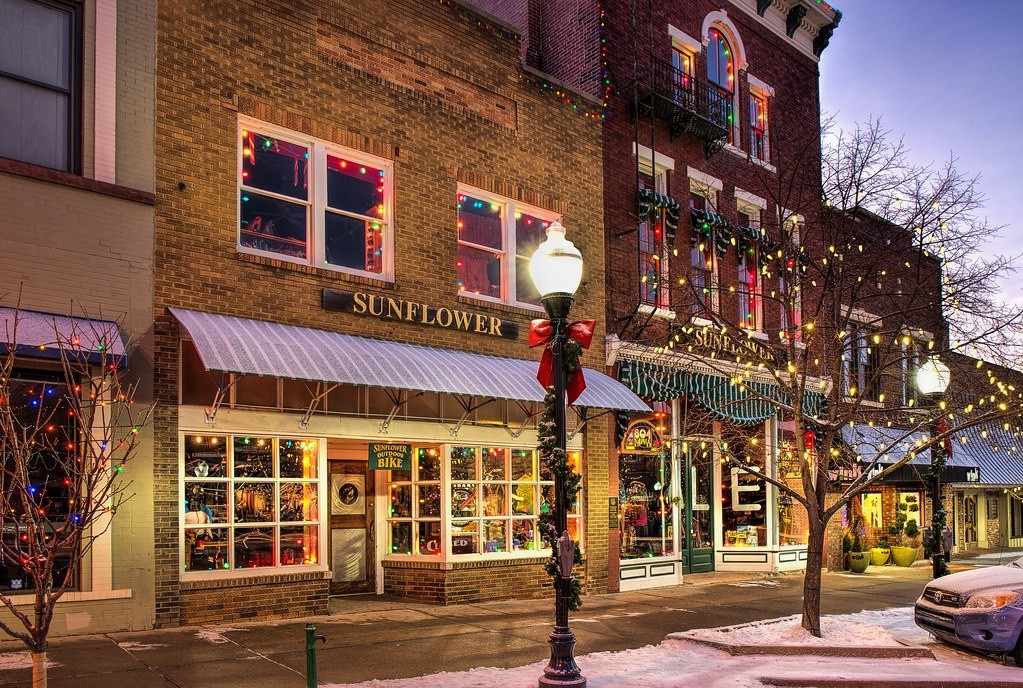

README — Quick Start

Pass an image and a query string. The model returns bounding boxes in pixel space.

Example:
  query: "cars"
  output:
[914,554,1022,670]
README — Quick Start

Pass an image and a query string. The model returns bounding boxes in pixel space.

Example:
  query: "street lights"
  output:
[918,347,951,579]
[529,221,588,688]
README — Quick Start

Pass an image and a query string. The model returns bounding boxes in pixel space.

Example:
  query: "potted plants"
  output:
[891,534,923,567]
[849,535,871,573]
[869,540,891,565]
